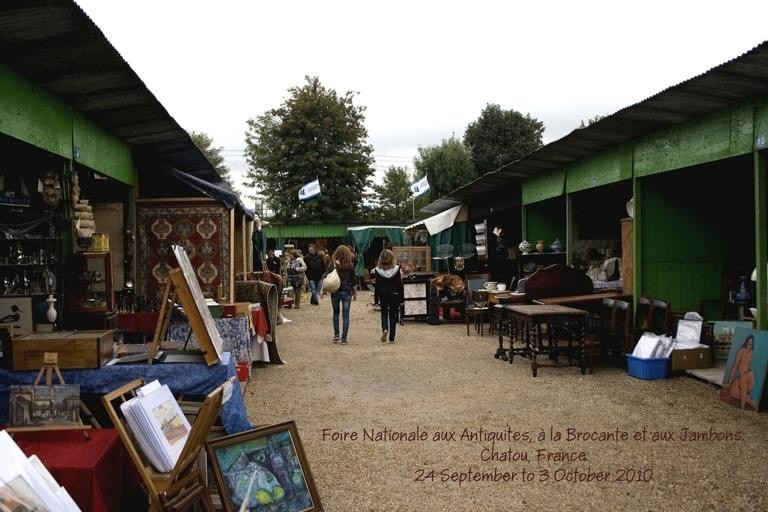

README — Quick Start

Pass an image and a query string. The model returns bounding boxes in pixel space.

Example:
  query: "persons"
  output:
[720,334,759,412]
[319,245,356,345]
[374,248,402,342]
[266,244,363,309]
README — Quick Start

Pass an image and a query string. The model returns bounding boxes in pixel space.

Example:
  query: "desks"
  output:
[12,426,143,511]
[170,317,255,366]
[1,352,253,433]
[504,304,590,376]
[486,288,528,337]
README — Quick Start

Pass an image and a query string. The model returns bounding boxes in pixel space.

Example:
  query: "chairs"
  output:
[580,298,673,363]
[463,290,492,336]
[69,308,119,331]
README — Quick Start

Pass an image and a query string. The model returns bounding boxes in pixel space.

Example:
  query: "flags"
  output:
[408,174,430,199]
[297,178,320,202]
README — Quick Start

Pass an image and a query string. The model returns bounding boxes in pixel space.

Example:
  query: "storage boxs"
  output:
[670,344,713,370]
[622,353,670,380]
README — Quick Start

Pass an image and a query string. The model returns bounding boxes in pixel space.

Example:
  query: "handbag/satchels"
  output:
[321,269,341,293]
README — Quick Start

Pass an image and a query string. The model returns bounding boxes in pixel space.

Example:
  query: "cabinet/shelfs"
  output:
[518,251,568,279]
[1,200,62,299]
[70,250,117,313]
[399,279,430,320]
[621,218,634,297]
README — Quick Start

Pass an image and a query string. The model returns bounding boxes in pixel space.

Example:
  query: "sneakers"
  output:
[284,297,319,309]
[333,335,340,341]
[341,340,348,345]
[381,329,388,343]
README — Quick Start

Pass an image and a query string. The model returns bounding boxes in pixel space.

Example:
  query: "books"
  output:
[120,377,192,474]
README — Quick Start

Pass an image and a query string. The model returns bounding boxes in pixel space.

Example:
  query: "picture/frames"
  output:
[207,419,324,512]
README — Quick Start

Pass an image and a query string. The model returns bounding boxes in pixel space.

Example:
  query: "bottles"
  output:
[625,195,634,218]
[518,238,562,256]
[735,274,750,302]
[46,295,58,323]
[496,283,507,291]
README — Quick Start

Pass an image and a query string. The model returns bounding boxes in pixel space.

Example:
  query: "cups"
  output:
[594,280,623,288]
[92,232,109,253]
[484,281,497,292]
